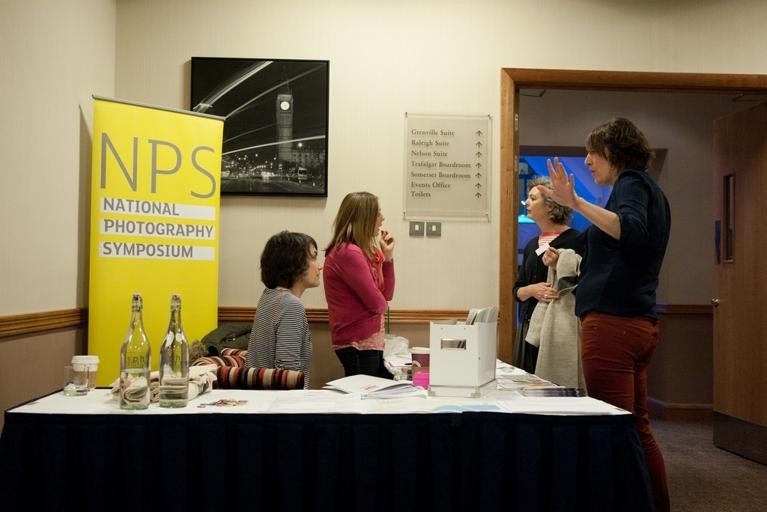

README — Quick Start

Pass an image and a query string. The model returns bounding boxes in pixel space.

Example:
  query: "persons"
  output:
[537,116,672,511]
[324,190,395,379]
[511,177,583,375]
[244,231,324,390]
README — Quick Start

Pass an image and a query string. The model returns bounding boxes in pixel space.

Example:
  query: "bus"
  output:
[287,167,308,181]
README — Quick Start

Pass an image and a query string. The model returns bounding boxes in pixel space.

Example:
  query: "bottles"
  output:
[158,293,191,409]
[118,293,153,411]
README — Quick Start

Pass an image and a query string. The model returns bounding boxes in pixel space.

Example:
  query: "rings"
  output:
[545,295,547,299]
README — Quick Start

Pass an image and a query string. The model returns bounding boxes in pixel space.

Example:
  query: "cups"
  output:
[62,354,99,397]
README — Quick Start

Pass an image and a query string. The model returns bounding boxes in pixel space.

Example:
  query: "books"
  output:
[321,368,418,395]
